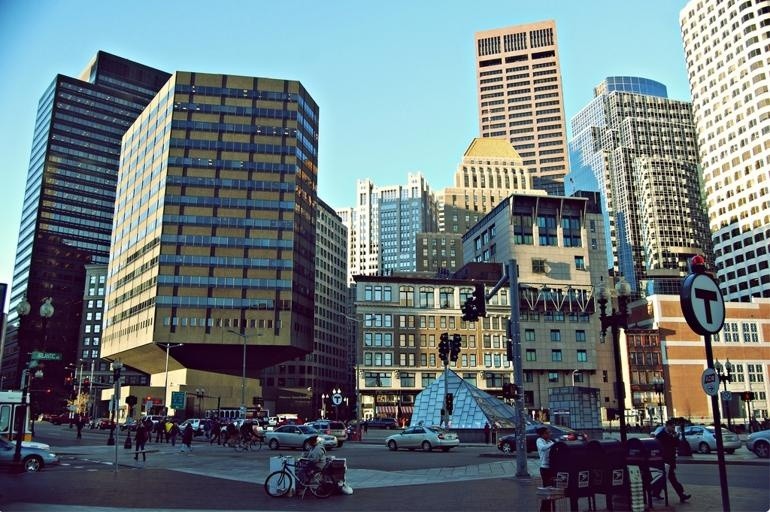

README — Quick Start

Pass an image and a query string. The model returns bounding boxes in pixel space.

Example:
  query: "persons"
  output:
[566,429,590,442]
[75,417,85,439]
[491,421,498,445]
[535,427,559,512]
[363,420,369,433]
[654,420,692,504]
[134,414,302,462]
[295,436,326,488]
[749,415,763,433]
[484,421,490,444]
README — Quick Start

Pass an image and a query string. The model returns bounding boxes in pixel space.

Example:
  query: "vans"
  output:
[360,417,398,429]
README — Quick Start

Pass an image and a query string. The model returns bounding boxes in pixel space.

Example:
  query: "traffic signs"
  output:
[30,351,63,361]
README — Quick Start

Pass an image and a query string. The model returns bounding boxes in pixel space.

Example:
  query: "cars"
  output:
[385,425,460,454]
[495,423,589,457]
[0,436,59,476]
[648,423,770,460]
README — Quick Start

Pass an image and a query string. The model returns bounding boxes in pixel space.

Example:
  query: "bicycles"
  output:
[264,456,337,499]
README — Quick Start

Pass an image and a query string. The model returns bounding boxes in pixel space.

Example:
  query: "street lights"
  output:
[64,358,96,425]
[152,340,184,417]
[595,276,632,459]
[742,392,755,431]
[320,387,348,422]
[653,370,666,427]
[715,356,734,432]
[13,291,59,472]
[227,329,264,415]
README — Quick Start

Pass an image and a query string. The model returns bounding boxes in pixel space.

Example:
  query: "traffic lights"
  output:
[447,393,454,411]
[460,297,478,322]
[471,284,486,317]
[438,332,462,362]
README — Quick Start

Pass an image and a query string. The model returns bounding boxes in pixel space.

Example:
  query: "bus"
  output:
[0,391,35,442]
